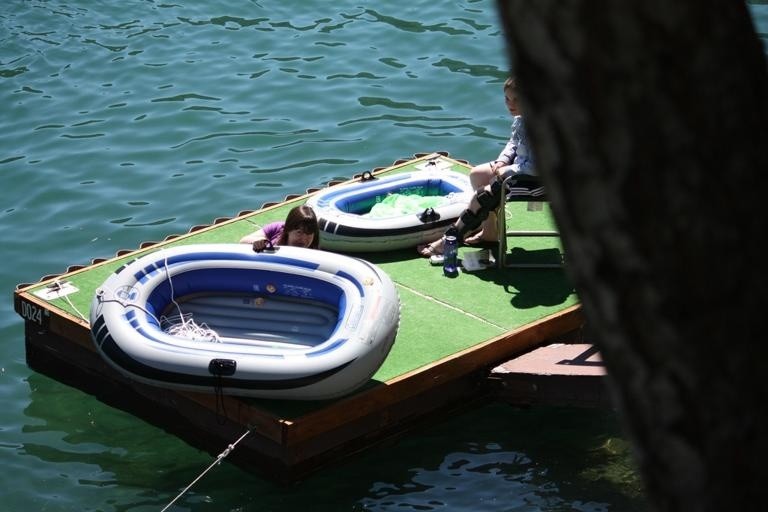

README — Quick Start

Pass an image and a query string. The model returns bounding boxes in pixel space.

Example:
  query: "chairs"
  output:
[497,172,568,270]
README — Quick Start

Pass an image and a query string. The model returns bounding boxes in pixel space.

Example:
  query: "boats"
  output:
[90,235,412,415]
[295,160,505,265]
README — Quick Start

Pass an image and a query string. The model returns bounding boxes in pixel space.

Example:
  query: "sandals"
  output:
[417,242,446,258]
[464,235,501,249]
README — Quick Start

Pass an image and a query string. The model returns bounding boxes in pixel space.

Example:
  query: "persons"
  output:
[416,74,553,258]
[239,205,320,252]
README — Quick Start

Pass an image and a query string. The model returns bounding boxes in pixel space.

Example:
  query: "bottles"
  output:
[444,237,458,272]
